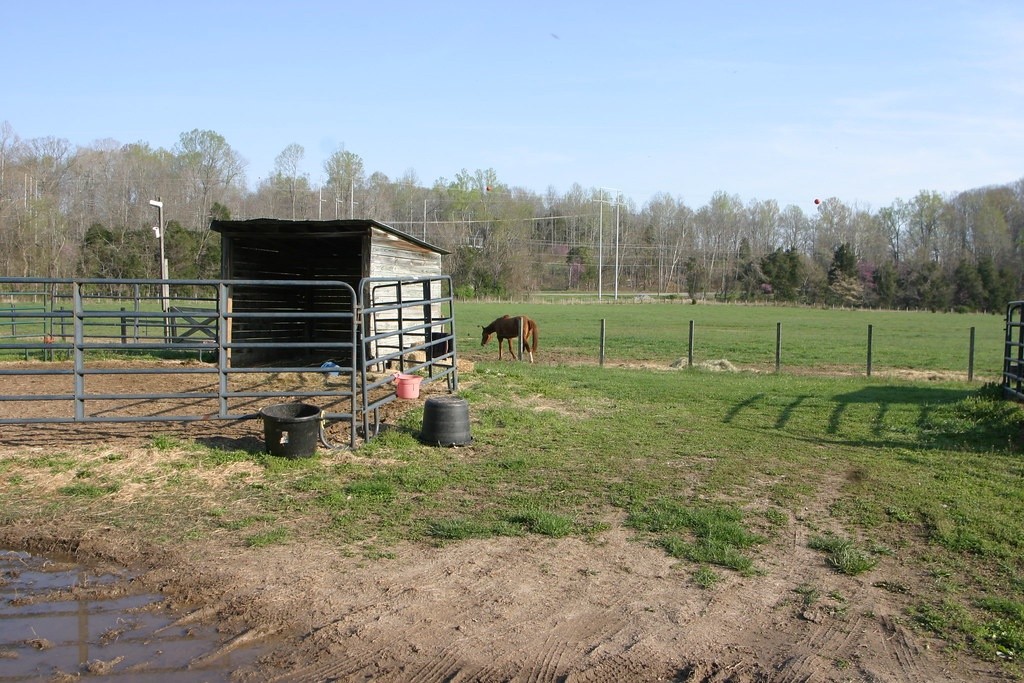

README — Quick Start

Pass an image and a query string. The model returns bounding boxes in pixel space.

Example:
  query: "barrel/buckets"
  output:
[260,404,321,458]
[395,375,423,400]
[418,395,472,446]
[320,362,340,376]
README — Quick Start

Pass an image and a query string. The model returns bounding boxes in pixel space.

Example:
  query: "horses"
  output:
[481,314,538,364]
